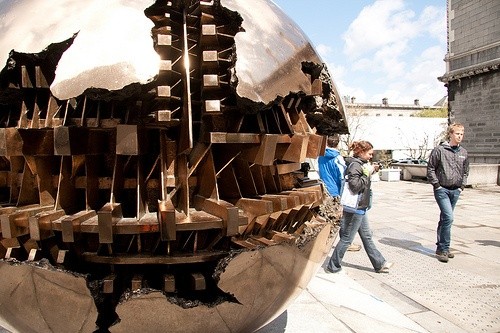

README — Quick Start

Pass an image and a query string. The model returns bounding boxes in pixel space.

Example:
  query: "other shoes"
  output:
[448,251,454,257]
[347,245,361,251]
[380,262,393,272]
[436,252,449,262]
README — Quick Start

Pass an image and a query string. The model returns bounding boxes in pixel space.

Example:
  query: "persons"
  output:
[427,123,470,261]
[327,141,394,274]
[318,134,362,251]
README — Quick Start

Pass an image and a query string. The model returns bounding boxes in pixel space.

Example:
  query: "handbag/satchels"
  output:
[340,162,362,212]
